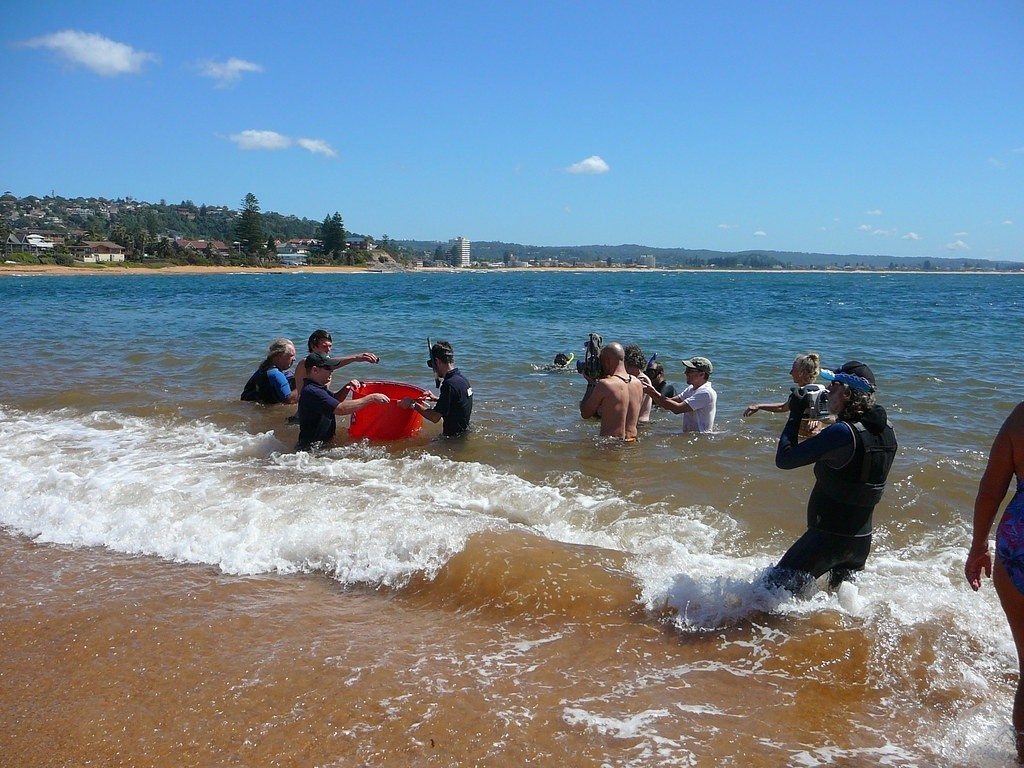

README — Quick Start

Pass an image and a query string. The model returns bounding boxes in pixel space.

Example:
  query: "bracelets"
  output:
[970,543,989,554]
[410,402,415,409]
[752,404,758,408]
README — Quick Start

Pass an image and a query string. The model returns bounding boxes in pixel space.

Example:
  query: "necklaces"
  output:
[613,374,631,383]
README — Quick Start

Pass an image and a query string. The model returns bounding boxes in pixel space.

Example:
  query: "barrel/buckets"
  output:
[350,380,428,441]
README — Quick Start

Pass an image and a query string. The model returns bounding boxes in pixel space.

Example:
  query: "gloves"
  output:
[785,388,809,428]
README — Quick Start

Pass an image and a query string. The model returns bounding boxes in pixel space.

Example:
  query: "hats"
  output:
[682,357,712,374]
[821,361,877,393]
[304,352,341,369]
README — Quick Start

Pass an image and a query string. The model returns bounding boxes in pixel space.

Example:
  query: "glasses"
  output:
[643,368,661,379]
[427,360,433,368]
[686,369,701,374]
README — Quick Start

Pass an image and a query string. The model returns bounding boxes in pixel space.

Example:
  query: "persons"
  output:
[399,341,473,442]
[294,330,378,400]
[643,362,675,407]
[964,399,1024,765]
[555,353,579,372]
[742,352,837,440]
[581,342,643,441]
[767,362,898,597]
[241,338,298,404]
[623,346,653,428]
[296,354,391,451]
[641,356,718,433]
